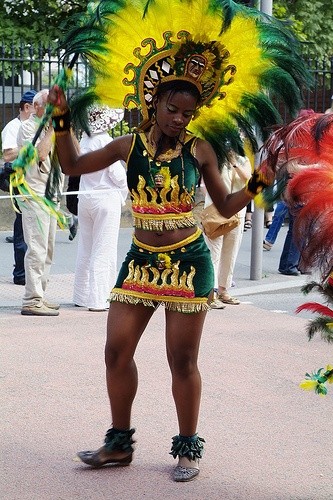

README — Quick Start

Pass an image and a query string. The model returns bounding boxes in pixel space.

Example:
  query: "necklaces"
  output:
[147,126,185,214]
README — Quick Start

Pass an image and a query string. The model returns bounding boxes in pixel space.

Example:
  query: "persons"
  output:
[202,137,253,311]
[5,174,81,243]
[16,89,64,316]
[72,101,129,311]
[244,108,331,278]
[2,91,36,285]
[46,78,279,482]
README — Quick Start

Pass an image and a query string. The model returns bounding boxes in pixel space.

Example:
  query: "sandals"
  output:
[217,291,240,304]
[210,299,227,309]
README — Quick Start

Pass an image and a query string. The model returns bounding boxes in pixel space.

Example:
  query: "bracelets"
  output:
[51,108,72,136]
[233,162,239,168]
[245,166,270,199]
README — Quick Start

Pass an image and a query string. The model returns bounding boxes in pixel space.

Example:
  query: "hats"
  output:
[22,90,38,102]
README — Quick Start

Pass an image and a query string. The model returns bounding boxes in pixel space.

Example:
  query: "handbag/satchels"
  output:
[199,200,241,239]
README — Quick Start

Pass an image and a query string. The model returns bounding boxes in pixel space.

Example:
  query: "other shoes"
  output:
[78,447,134,468]
[43,300,61,309]
[171,455,199,482]
[68,223,77,241]
[6,236,14,243]
[289,271,300,276]
[21,302,60,316]
